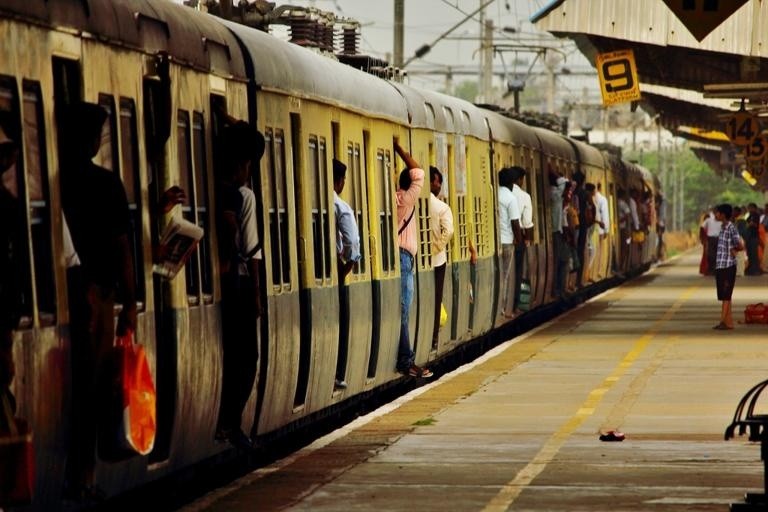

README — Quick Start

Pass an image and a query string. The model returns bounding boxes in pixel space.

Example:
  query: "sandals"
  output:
[407,364,434,378]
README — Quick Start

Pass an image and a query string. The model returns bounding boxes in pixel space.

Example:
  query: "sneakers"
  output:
[225,426,252,451]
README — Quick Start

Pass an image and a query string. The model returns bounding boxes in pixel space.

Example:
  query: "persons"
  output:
[699,202,768,275]
[393,140,433,378]
[56,107,137,508]
[1,142,34,432]
[210,115,263,450]
[498,166,666,317]
[332,158,361,389]
[713,205,745,330]
[158,186,186,214]
[429,165,454,350]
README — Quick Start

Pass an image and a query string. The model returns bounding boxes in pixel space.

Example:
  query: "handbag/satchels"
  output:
[744,303,768,324]
[114,330,157,456]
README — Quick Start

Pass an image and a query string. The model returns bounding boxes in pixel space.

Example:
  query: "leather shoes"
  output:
[336,382,348,389]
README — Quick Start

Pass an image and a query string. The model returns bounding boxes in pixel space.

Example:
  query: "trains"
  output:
[0,0,663,512]
